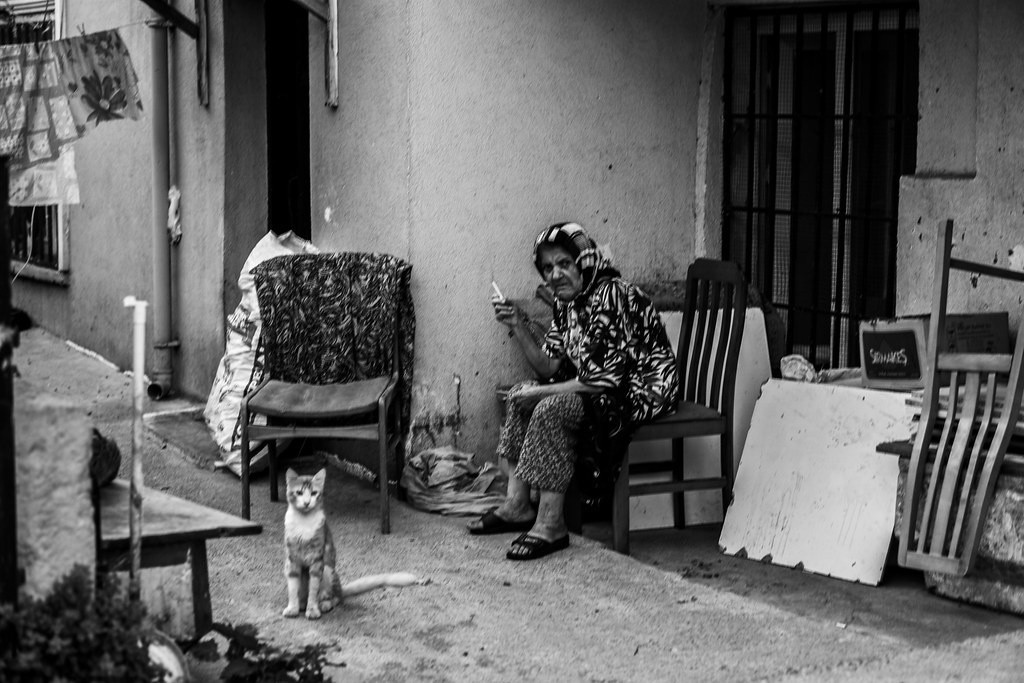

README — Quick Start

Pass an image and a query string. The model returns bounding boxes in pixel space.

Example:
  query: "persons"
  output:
[465,223,678,562]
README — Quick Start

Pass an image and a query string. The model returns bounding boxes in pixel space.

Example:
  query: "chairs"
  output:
[898,220,1024,576]
[602,257,749,552]
[241,253,413,533]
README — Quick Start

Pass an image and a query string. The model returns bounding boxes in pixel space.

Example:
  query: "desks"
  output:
[100,476,263,647]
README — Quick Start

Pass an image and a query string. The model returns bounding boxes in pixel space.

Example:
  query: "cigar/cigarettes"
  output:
[491,280,506,304]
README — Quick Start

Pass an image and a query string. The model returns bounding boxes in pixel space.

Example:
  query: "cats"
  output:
[282,468,419,620]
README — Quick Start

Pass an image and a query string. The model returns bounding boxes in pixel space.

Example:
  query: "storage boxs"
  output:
[858,311,1009,390]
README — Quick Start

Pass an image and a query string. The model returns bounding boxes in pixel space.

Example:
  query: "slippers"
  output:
[505,531,570,561]
[465,508,537,535]
